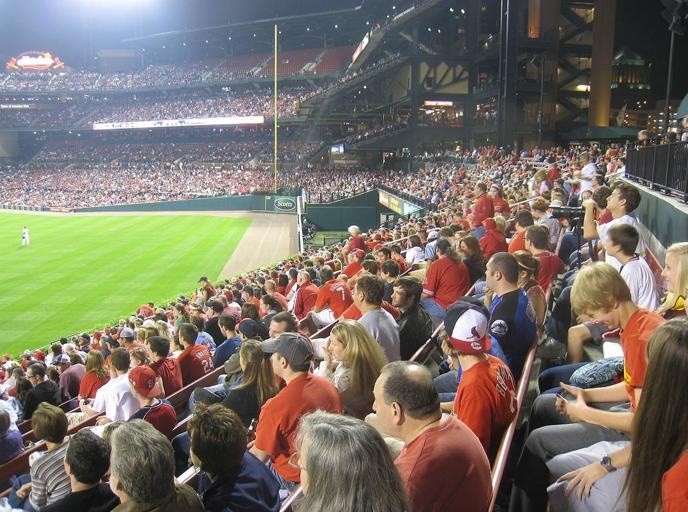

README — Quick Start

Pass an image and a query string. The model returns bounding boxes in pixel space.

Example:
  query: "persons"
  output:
[22,225,31,248]
[1,259,686,512]
[0,47,399,214]
[302,103,688,259]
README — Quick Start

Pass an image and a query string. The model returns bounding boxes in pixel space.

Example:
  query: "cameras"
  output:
[549,204,597,231]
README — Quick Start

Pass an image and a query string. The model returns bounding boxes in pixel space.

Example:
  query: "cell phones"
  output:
[557,388,576,401]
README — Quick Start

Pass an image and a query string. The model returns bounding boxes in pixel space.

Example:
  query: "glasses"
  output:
[287,452,307,470]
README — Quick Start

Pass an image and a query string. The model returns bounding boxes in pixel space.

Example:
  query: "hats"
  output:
[428,231,438,239]
[553,178,563,184]
[352,250,365,258]
[444,297,491,354]
[0,273,315,396]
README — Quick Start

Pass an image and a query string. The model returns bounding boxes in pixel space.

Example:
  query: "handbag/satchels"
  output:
[568,356,625,387]
